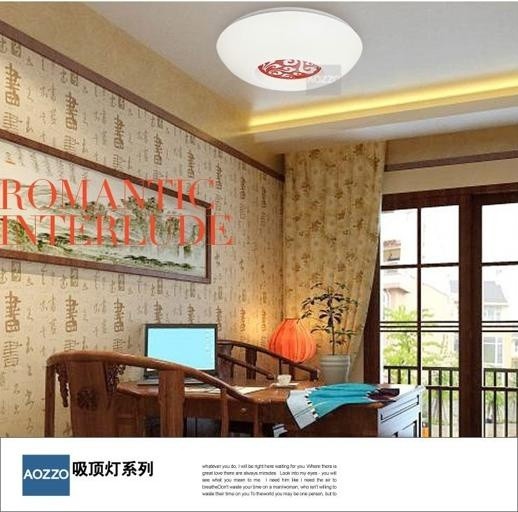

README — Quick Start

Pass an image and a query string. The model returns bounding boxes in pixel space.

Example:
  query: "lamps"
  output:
[215,7,363,92]
[268,317,317,380]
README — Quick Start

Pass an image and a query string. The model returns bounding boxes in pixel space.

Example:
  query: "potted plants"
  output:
[301,282,361,383]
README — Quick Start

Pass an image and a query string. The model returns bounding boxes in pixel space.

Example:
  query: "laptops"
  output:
[137,323,217,387]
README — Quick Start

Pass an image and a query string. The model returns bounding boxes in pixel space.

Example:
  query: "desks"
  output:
[118,381,427,437]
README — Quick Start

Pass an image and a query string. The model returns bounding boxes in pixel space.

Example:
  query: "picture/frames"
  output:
[0,129,211,284]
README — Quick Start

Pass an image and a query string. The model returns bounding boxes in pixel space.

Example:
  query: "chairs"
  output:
[45,351,271,437]
[215,339,318,438]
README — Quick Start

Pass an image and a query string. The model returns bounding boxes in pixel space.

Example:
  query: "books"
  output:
[210,386,268,395]
[185,385,215,394]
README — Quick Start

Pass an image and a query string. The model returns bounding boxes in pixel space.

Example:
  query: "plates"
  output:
[267,382,300,389]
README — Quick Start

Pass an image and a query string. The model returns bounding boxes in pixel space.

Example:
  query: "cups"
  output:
[278,373,293,384]
